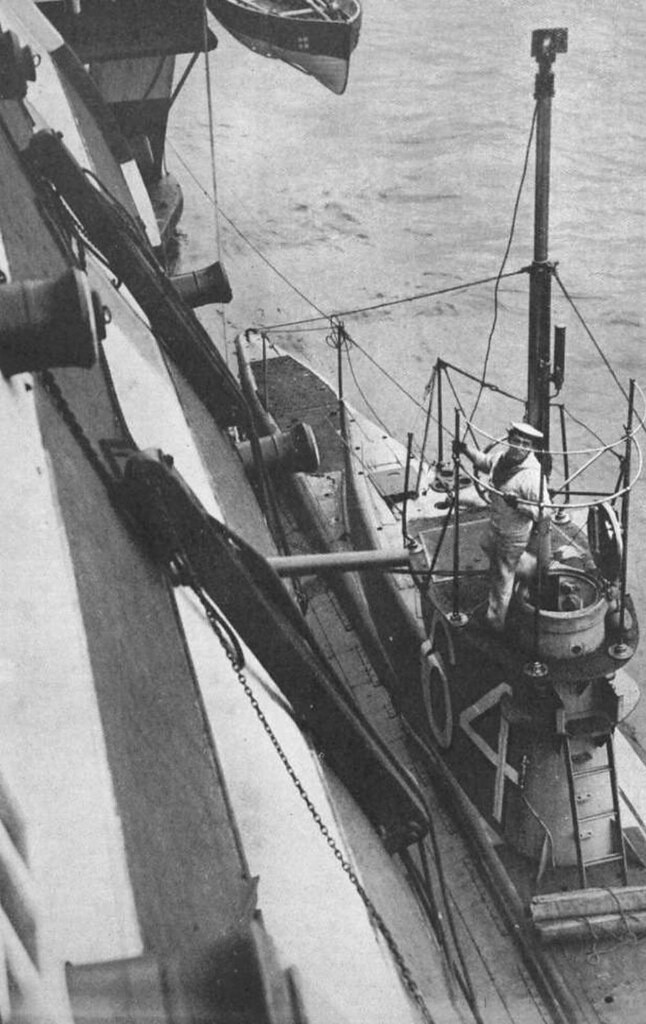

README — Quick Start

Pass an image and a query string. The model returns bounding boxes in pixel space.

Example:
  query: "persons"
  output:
[452,422,554,630]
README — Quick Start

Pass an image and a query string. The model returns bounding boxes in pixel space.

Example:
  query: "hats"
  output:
[509,421,544,443]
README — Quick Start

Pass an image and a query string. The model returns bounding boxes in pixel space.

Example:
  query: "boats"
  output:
[207,1,362,59]
[233,27,646,1023]
